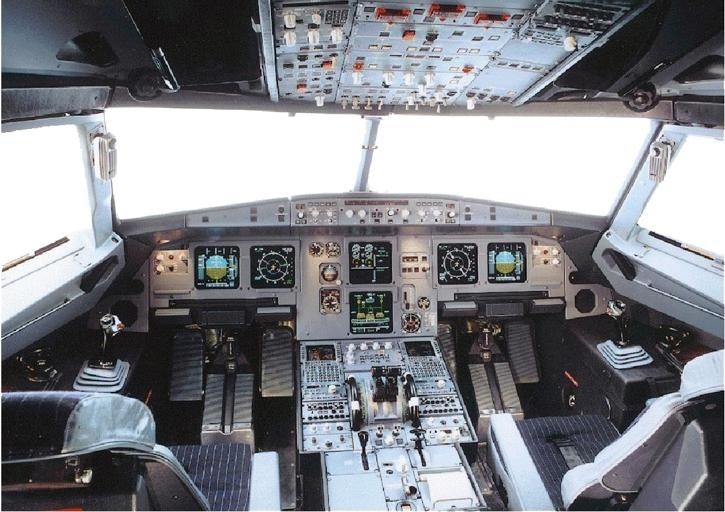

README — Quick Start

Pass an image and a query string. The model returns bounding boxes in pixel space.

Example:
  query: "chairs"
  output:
[485,349,724,510]
[2,390,280,510]
